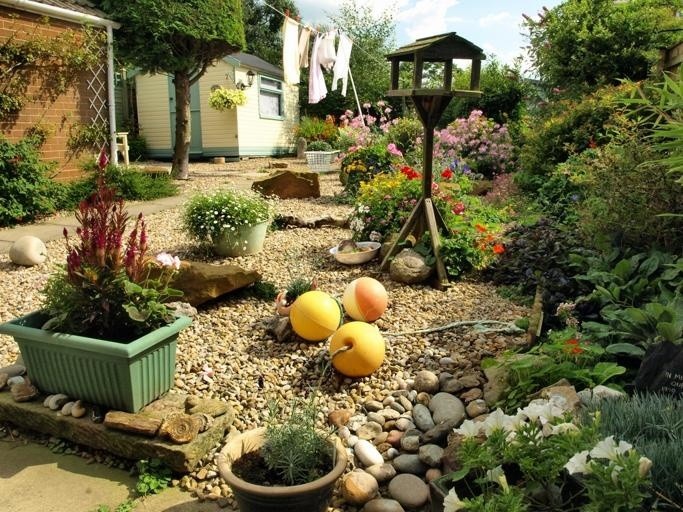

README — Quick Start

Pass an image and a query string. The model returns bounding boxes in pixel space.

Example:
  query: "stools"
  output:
[114,132,130,165]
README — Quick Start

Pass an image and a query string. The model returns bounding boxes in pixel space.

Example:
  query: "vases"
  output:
[427,458,592,512]
[0,308,192,414]
[213,217,269,256]
[329,240,381,264]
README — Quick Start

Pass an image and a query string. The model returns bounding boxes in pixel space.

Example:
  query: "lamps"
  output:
[240,70,255,90]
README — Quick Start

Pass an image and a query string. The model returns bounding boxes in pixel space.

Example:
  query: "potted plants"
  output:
[214,379,348,510]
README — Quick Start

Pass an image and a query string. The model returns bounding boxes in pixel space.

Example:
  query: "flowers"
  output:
[439,391,658,512]
[179,185,269,257]
[39,144,184,343]
[336,199,370,252]
